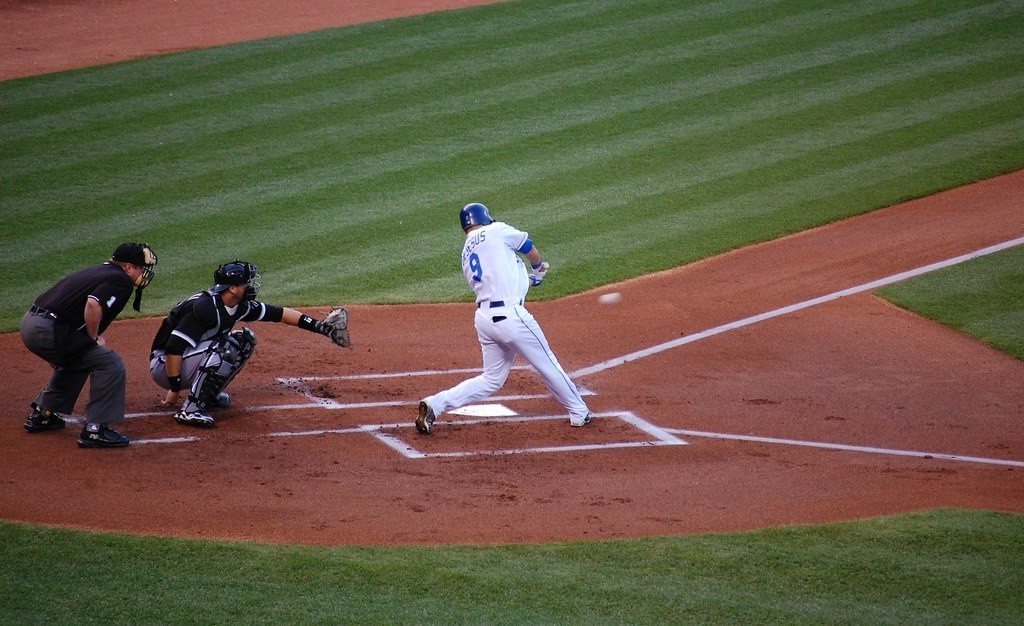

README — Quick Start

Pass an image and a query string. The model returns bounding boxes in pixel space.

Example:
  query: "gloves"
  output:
[529,273,543,286]
[531,261,547,278]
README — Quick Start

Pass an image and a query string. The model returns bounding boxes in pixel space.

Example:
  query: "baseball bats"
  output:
[539,262,549,272]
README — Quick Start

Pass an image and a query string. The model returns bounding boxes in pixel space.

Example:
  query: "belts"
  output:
[31,305,53,319]
[477,300,523,309]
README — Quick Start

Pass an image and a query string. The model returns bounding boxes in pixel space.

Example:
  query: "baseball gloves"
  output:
[323,306,353,350]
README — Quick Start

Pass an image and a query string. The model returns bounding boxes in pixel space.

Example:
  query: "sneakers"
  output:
[415,398,435,434]
[174,409,214,427]
[23,406,66,433]
[212,392,231,408]
[77,423,129,449]
[571,410,592,428]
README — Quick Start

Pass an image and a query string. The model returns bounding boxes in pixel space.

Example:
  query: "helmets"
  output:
[210,260,256,293]
[460,202,494,230]
[112,242,157,266]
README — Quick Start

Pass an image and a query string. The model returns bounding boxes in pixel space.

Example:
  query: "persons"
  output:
[416,203,592,434]
[149,261,349,427]
[20,243,158,448]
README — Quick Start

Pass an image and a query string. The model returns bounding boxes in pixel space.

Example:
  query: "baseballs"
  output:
[599,291,623,306]
